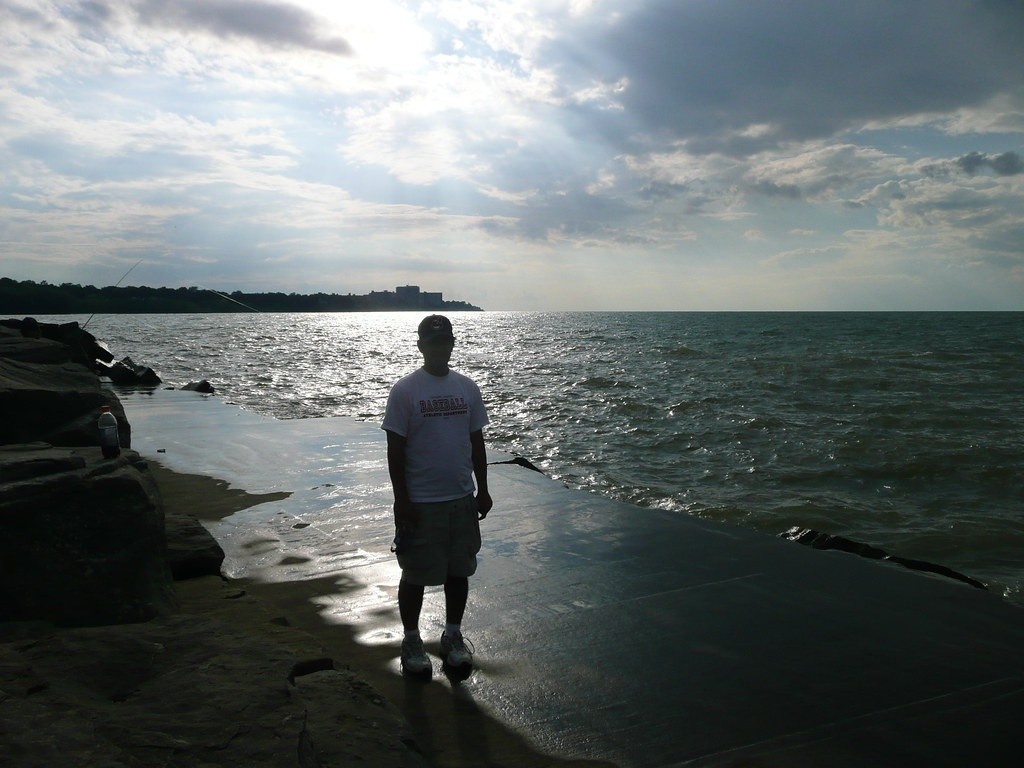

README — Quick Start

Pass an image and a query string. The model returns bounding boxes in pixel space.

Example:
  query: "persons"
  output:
[381,314,492,674]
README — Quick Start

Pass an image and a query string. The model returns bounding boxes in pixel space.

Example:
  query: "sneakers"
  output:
[439,631,475,671]
[398,636,434,678]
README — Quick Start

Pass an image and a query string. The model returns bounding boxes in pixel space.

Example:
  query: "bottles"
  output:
[97,406,121,459]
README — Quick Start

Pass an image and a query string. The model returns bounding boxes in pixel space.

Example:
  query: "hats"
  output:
[417,314,457,342]
